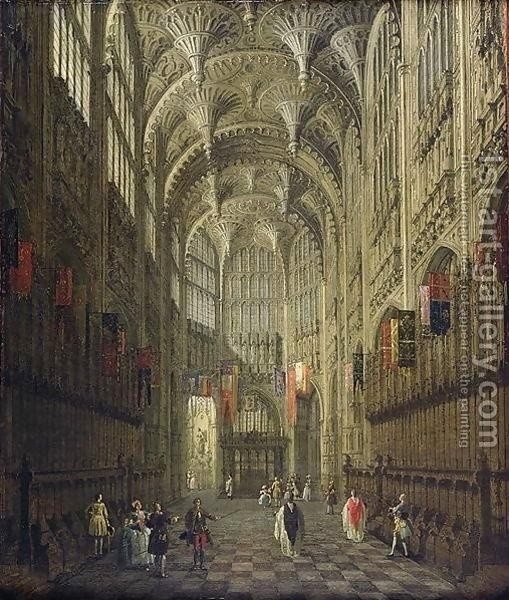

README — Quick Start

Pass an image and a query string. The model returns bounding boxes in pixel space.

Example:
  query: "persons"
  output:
[259,485,272,509]
[282,485,291,499]
[341,489,366,543]
[274,493,305,558]
[84,492,110,557]
[325,480,336,515]
[302,474,312,503]
[384,493,411,559]
[184,497,222,571]
[226,475,233,501]
[190,470,200,489]
[122,499,154,572]
[291,480,300,499]
[272,477,282,508]
[187,471,191,489]
[145,501,180,578]
